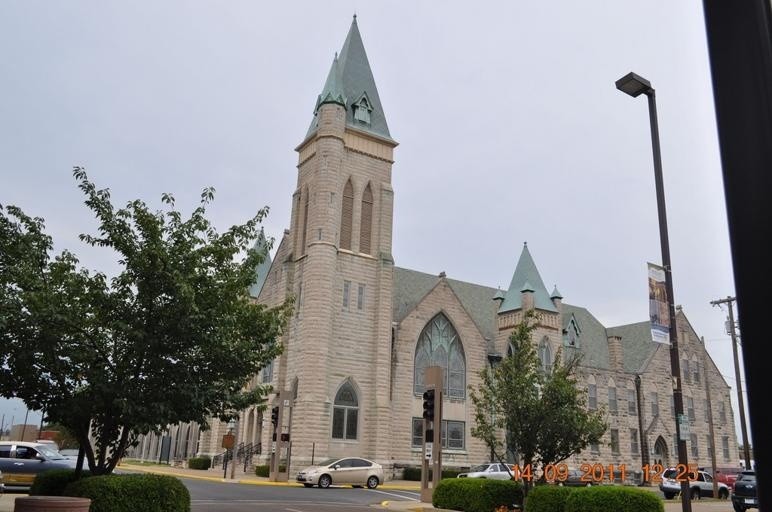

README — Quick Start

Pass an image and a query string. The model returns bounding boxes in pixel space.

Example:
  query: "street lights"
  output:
[630,371,655,487]
[614,71,695,512]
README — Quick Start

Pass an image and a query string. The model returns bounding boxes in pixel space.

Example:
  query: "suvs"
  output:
[699,465,745,492]
[658,465,729,501]
[455,460,526,483]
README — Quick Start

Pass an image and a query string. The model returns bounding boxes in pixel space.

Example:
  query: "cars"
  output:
[0,440,117,493]
[58,447,92,462]
[296,456,385,490]
[532,466,595,488]
[731,470,759,512]
[37,438,61,459]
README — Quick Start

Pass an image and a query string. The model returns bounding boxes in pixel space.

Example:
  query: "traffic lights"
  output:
[272,406,277,426]
[422,387,435,420]
[281,434,290,442]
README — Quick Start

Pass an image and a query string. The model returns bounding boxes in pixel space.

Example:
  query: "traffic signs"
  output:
[424,442,434,461]
[271,441,276,454]
